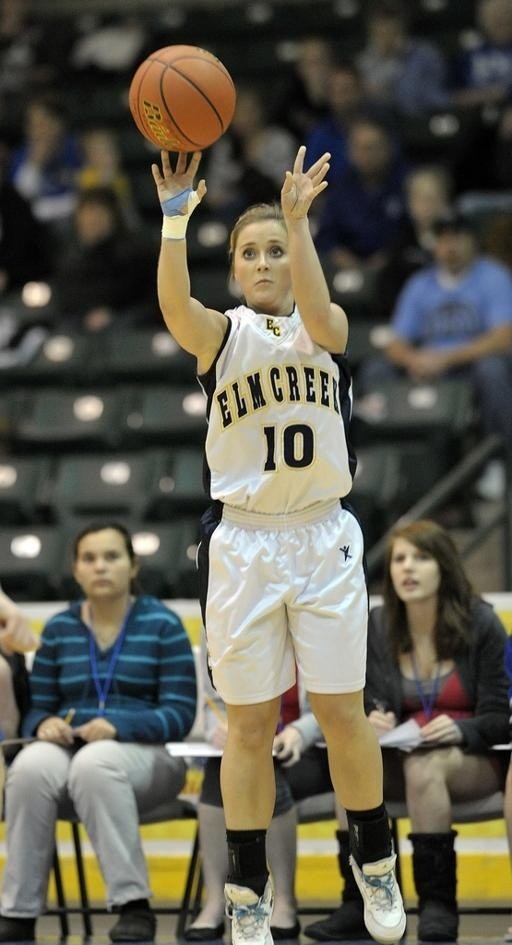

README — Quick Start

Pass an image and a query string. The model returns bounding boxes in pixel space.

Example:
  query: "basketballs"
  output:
[129,44,236,152]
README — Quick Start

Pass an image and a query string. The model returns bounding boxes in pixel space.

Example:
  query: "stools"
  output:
[375,789,512,945]
[179,794,338,940]
[47,798,201,941]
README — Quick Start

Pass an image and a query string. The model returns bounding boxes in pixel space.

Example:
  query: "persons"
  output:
[2,589,43,820]
[301,518,511,944]
[1,519,198,945]
[186,515,335,945]
[0,0,511,501]
[149,142,408,945]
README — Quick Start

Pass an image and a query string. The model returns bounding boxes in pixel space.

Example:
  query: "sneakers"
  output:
[110,908,156,941]
[273,917,300,938]
[223,860,275,945]
[349,837,406,945]
[0,918,35,943]
[184,921,224,942]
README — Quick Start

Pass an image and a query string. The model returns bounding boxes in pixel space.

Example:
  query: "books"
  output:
[313,716,428,755]
[162,738,281,759]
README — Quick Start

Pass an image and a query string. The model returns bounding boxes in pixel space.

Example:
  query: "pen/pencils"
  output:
[62,709,78,726]
[370,695,389,716]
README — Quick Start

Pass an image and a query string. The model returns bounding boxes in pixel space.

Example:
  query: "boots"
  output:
[304,829,371,941]
[407,830,458,942]
